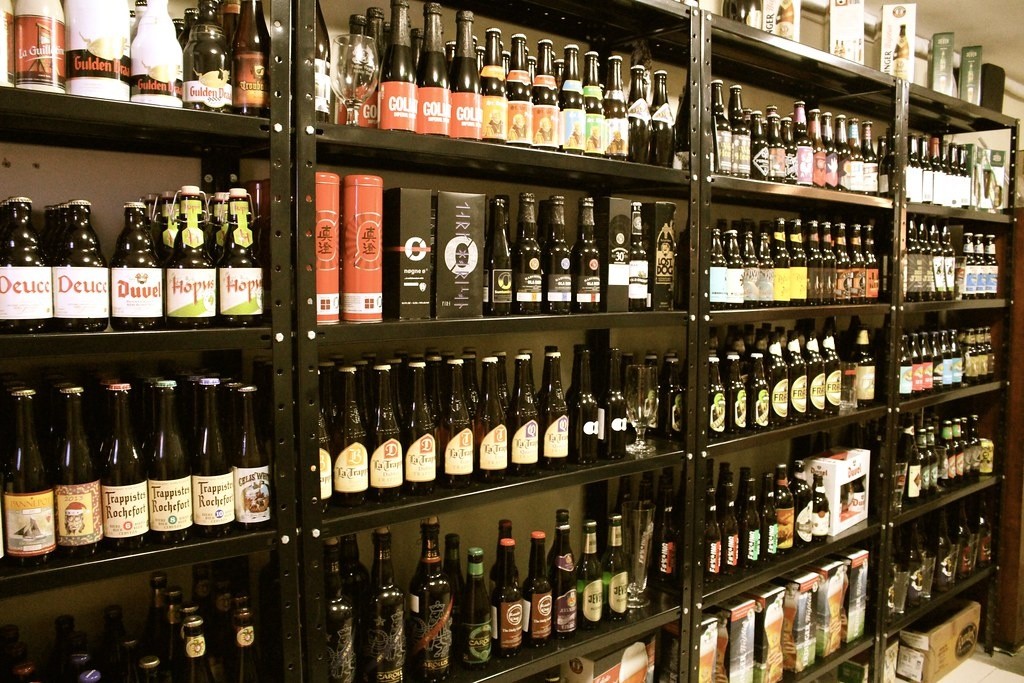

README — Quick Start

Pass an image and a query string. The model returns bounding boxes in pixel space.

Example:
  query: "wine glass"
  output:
[935,446,948,492]
[624,364,660,456]
[621,501,655,609]
[330,34,379,128]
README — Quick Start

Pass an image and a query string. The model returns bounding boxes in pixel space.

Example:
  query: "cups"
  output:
[948,544,960,584]
[922,553,936,598]
[893,567,910,614]
[839,362,859,410]
[970,534,980,569]
[894,460,908,507]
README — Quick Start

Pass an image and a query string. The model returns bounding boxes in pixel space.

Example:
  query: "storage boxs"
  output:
[593,196,630,313]
[640,202,677,312]
[758,1,981,103]
[559,640,649,682]
[804,446,871,537]
[698,548,898,683]
[432,191,485,320]
[382,187,434,321]
[896,598,981,682]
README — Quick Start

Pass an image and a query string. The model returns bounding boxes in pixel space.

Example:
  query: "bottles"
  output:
[776,0,794,40]
[0,0,1011,683]
[893,25,909,80]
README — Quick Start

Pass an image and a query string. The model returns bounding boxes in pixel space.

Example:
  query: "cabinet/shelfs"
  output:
[0,1,302,683]
[690,1,882,683]
[296,0,688,683]
[881,73,1023,683]
